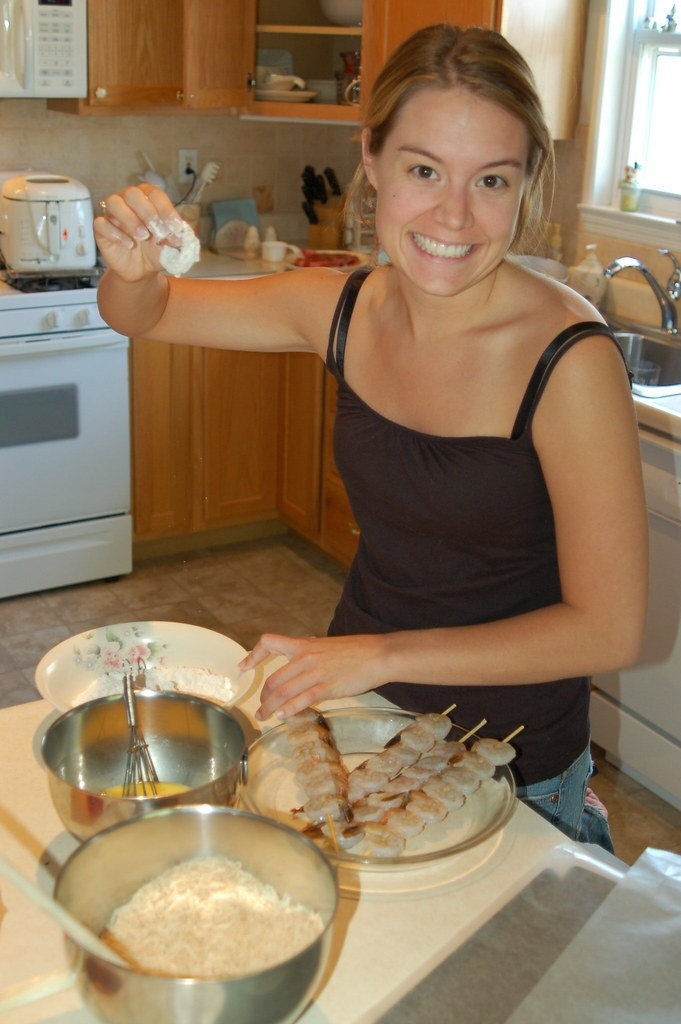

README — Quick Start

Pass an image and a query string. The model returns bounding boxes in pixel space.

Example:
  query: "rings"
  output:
[98,199,107,216]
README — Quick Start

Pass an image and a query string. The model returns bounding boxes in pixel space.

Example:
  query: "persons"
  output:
[95,22,649,856]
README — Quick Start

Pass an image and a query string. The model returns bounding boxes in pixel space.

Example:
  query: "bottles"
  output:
[339,50,359,105]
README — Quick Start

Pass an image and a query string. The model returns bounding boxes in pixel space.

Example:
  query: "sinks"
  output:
[605,313,681,399]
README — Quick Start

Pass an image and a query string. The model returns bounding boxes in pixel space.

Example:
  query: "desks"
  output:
[0,647,681,1024]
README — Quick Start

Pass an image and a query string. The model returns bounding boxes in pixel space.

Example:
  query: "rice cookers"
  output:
[0,173,97,272]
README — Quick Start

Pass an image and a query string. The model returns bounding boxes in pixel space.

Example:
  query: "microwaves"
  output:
[0,0,90,98]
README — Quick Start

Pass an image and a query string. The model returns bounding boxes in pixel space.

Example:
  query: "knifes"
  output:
[301,164,342,225]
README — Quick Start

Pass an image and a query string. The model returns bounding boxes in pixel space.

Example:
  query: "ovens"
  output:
[0,329,134,600]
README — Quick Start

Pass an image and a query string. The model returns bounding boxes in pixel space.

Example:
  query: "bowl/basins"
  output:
[31,690,248,841]
[306,79,337,103]
[508,255,570,283]
[319,0,362,26]
[627,359,661,386]
[51,803,338,1024]
[255,65,296,90]
[35,621,255,713]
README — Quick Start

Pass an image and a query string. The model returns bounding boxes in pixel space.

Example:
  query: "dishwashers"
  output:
[587,428,681,812]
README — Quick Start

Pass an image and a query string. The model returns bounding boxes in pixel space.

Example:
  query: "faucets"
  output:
[602,247,681,334]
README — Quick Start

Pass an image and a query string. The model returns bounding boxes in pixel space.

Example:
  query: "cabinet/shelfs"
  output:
[279,352,360,579]
[46,0,503,131]
[127,320,286,563]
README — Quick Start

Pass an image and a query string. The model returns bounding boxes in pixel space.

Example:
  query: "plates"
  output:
[235,705,518,875]
[282,249,371,272]
[255,89,319,103]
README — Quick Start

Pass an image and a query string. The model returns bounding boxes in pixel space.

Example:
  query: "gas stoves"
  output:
[0,258,110,337]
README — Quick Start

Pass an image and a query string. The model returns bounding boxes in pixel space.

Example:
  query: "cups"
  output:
[262,241,287,262]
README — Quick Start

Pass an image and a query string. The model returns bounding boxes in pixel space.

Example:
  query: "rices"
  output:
[98,854,327,980]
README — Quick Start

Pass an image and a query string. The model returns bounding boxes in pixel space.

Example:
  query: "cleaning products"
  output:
[551,222,563,264]
[572,244,606,313]
